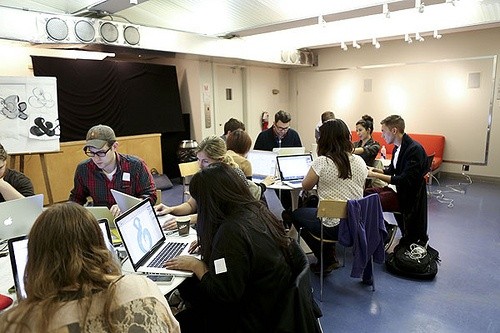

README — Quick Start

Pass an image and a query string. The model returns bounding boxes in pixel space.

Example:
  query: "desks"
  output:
[249,164,378,212]
[0,209,204,333]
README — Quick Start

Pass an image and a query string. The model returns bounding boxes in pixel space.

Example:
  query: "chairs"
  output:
[179,160,202,204]
[287,237,324,333]
[387,161,432,246]
[296,192,389,300]
[422,153,436,200]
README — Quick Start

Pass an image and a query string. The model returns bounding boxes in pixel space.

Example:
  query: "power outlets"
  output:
[462,164,470,172]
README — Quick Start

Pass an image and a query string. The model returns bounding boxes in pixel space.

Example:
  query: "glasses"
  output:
[85,146,112,158]
[275,123,290,131]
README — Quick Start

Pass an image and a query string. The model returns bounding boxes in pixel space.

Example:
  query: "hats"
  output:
[83,124,116,151]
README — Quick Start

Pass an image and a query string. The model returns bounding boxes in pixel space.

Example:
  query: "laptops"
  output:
[114,197,202,278]
[8,218,113,304]
[273,147,305,177]
[247,150,276,182]
[110,189,177,227]
[0,194,43,238]
[84,207,116,228]
[277,152,314,189]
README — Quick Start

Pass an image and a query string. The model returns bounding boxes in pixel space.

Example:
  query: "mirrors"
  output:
[122,24,141,46]
[44,16,71,42]
[99,20,120,44]
[73,18,96,43]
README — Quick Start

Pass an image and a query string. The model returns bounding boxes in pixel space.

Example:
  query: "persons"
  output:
[163,161,293,333]
[154,135,249,231]
[226,130,277,197]
[254,109,305,211]
[363,113,429,252]
[350,114,380,163]
[66,124,157,218]
[220,118,246,144]
[0,143,35,202]
[0,200,183,333]
[313,111,352,146]
[291,118,368,275]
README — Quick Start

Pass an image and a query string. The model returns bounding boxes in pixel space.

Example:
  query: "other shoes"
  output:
[384,224,397,251]
[310,256,339,273]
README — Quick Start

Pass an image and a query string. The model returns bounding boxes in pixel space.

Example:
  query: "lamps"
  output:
[383,4,391,19]
[352,41,361,50]
[341,42,348,50]
[372,37,380,48]
[317,15,326,28]
[414,0,425,13]
[434,29,443,40]
[415,32,425,42]
[405,34,412,44]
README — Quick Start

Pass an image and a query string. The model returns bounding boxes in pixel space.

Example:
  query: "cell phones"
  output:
[146,275,175,284]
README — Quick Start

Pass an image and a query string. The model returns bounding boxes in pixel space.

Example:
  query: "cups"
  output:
[175,217,191,237]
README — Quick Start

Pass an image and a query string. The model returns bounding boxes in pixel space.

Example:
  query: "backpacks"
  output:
[385,238,441,279]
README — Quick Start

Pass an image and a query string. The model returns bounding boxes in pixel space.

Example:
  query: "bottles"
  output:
[381,145,386,161]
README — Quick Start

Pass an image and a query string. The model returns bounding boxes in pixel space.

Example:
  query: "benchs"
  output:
[350,130,445,185]
